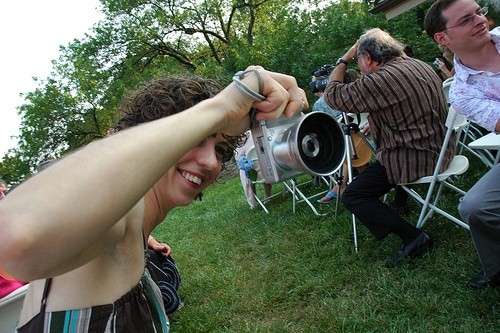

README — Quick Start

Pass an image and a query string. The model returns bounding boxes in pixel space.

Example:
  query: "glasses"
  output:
[353,53,363,65]
[442,7,489,30]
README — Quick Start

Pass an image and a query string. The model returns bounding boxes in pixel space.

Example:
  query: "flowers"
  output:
[238,156,258,185]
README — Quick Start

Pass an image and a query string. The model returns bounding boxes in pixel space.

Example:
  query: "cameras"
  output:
[433,60,441,70]
[251,108,346,184]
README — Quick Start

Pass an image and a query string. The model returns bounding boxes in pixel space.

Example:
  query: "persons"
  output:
[234,0,500,290]
[0,65,309,333]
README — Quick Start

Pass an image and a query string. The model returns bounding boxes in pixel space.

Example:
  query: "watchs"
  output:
[336,58,348,67]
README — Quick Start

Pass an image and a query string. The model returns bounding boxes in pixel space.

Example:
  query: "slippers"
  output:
[317,190,348,204]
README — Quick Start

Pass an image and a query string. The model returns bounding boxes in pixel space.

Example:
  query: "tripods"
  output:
[334,111,379,256]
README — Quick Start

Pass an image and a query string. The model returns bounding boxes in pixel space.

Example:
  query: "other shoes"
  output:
[468,271,500,290]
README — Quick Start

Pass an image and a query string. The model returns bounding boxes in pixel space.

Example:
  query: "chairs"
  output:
[337,112,369,129]
[397,105,470,231]
[443,81,495,168]
[245,147,286,214]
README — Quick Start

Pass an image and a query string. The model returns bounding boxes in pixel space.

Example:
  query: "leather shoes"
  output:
[386,232,432,269]
